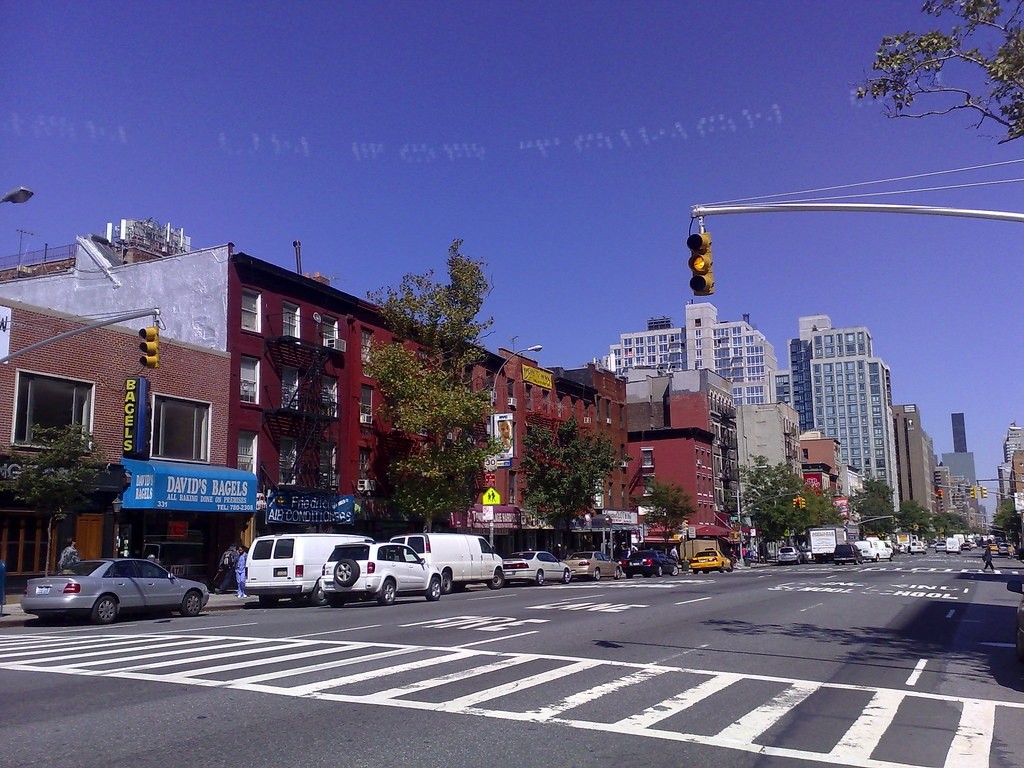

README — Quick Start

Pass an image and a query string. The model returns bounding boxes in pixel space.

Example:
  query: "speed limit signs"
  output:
[484,455,498,472]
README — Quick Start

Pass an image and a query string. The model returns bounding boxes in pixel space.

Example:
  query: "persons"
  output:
[233,545,247,598]
[220,543,238,594]
[742,545,747,557]
[620,542,629,559]
[730,546,737,568]
[1008,545,1014,558]
[781,541,787,546]
[982,544,995,573]
[58,536,79,568]
[496,420,513,446]
[670,546,678,559]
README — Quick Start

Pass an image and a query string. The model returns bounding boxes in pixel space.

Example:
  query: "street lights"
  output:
[736,465,769,562]
[997,465,1024,550]
[489,343,543,549]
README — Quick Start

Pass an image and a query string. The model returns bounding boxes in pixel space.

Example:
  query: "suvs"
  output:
[318,539,444,608]
[834,543,864,565]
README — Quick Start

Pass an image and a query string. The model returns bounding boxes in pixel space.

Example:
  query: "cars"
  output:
[689,548,734,575]
[562,551,623,581]
[501,550,572,586]
[778,546,802,564]
[885,534,1009,556]
[1006,579,1024,664]
[19,558,209,625]
[623,550,680,580]
[800,549,812,562]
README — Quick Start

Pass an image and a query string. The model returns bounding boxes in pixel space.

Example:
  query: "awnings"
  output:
[644,536,681,543]
[119,458,257,512]
[449,504,521,528]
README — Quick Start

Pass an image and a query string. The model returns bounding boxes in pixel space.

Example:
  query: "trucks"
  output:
[810,528,846,564]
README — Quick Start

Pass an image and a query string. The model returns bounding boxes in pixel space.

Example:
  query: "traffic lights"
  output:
[970,485,976,499]
[686,232,715,297]
[138,326,159,369]
[980,485,988,498]
[683,523,688,535]
[801,498,806,508]
[792,498,797,508]
[938,489,942,500]
[796,499,801,509]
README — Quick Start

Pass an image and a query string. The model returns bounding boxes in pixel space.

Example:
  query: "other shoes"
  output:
[243,594,247,597]
[237,594,242,598]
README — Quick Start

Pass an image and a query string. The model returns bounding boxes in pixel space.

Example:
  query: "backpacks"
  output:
[221,553,234,567]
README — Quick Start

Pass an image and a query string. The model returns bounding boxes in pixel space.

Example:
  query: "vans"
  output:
[854,541,893,562]
[386,532,505,594]
[244,533,385,608]
